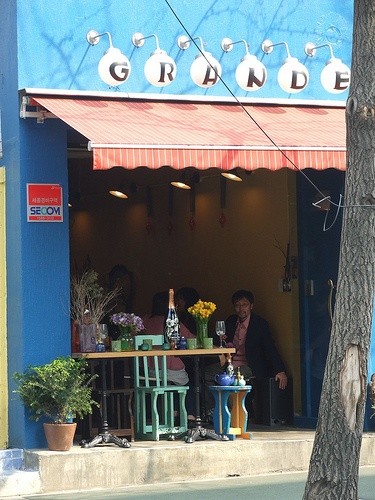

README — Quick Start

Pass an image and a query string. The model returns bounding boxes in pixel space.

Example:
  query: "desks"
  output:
[71,347,237,449]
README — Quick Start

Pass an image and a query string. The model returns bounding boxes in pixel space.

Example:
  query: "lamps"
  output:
[132,32,178,88]
[177,35,223,89]
[261,39,309,93]
[107,181,137,201]
[170,171,200,190]
[86,30,131,87]
[219,169,253,183]
[304,40,352,95]
[220,37,268,91]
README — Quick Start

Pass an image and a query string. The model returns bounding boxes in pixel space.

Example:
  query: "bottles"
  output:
[164,288,181,350]
[180,337,187,350]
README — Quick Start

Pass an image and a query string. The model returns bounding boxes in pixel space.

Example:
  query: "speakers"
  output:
[252,375,294,426]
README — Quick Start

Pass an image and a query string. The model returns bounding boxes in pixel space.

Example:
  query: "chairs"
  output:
[89,358,137,442]
[133,334,188,442]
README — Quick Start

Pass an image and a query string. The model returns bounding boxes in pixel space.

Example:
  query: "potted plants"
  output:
[8,352,101,453]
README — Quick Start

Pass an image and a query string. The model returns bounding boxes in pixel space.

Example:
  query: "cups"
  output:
[187,338,197,349]
[143,339,152,351]
[203,337,213,349]
[111,341,121,352]
[96,324,108,339]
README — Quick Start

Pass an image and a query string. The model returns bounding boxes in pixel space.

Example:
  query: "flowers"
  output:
[187,299,217,344]
[109,312,146,342]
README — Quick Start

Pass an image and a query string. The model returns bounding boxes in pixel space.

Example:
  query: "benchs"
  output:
[189,375,295,427]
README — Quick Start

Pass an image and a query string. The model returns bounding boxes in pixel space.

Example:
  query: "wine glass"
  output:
[216,320,226,349]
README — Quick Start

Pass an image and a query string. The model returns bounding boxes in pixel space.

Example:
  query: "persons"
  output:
[203,290,289,425]
[99,265,215,427]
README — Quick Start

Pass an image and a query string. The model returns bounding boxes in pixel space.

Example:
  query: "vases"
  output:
[110,339,132,352]
[118,325,134,351]
[196,323,209,349]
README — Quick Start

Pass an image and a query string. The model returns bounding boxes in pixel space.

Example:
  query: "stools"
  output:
[207,385,253,441]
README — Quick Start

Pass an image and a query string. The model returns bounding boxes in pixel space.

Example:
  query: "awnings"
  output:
[23,95,347,173]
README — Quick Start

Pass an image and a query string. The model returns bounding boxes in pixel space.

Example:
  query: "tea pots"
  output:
[214,372,236,386]
